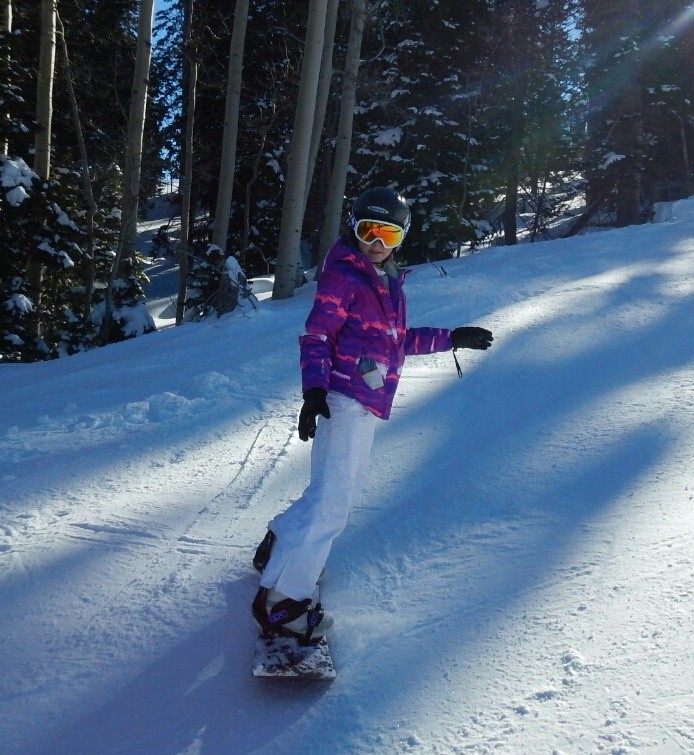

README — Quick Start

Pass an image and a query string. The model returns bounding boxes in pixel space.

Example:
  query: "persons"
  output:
[253,186,493,637]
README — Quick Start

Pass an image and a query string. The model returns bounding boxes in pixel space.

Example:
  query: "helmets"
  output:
[353,188,411,234]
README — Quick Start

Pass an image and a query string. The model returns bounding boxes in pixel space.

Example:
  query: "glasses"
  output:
[353,218,404,250]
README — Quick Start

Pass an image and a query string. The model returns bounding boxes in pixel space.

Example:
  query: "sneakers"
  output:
[266,599,334,636]
[317,567,326,581]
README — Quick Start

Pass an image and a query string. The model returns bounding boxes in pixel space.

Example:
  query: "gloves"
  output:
[452,327,493,351]
[297,387,332,442]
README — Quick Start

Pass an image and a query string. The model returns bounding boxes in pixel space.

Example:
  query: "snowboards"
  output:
[252,581,336,680]
